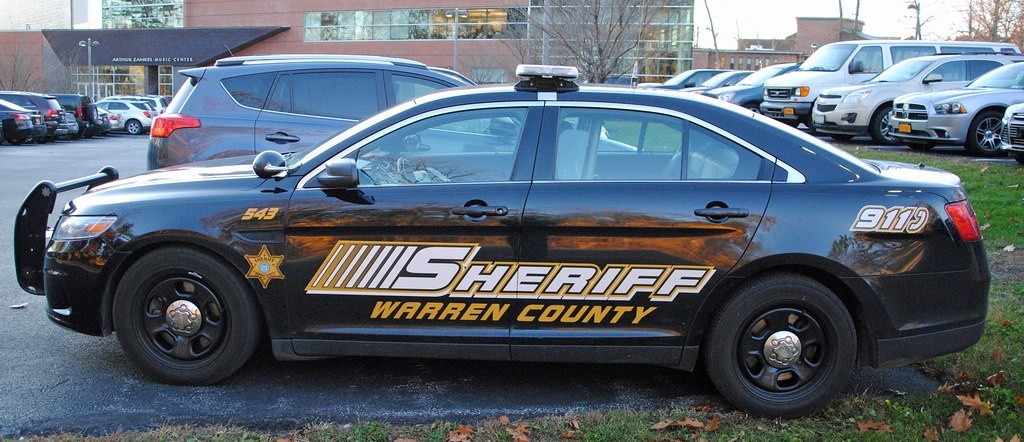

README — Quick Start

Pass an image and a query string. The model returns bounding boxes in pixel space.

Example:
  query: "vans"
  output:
[759,40,1021,123]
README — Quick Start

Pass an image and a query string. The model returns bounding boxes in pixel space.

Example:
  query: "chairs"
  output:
[554,129,590,180]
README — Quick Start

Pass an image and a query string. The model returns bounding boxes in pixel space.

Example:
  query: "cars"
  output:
[810,53,1023,159]
[92,95,167,134]
[11,65,991,421]
[635,64,802,109]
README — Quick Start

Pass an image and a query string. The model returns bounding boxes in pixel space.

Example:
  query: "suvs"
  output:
[0,90,110,143]
[147,52,642,172]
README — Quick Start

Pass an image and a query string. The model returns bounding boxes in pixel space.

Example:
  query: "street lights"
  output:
[79,36,99,99]
